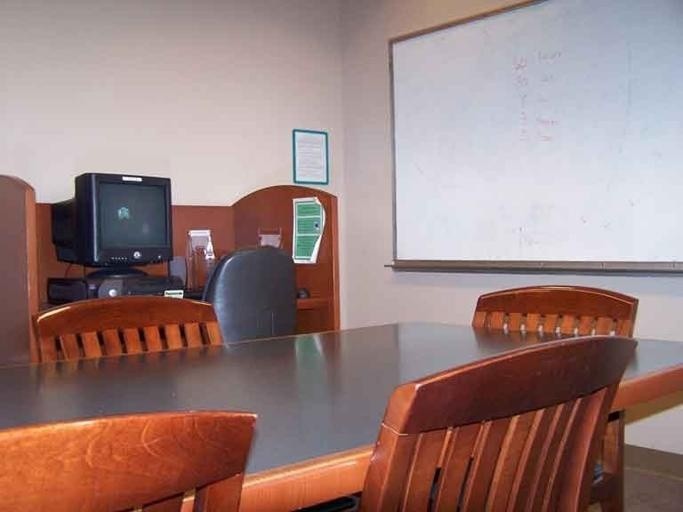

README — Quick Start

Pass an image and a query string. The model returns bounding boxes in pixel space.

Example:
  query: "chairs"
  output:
[202,245,298,344]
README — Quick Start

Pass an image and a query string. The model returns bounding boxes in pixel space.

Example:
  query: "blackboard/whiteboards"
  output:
[388,0,683,275]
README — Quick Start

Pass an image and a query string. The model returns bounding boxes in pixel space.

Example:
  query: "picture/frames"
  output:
[292,129,328,184]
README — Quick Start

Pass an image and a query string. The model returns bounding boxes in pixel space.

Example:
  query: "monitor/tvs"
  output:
[50,173,173,277]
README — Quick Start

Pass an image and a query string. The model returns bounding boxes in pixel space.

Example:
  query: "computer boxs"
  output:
[47,277,184,303]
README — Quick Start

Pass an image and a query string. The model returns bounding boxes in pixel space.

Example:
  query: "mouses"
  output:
[297,287,310,299]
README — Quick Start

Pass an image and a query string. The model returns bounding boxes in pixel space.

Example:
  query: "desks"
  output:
[1,174,341,349]
